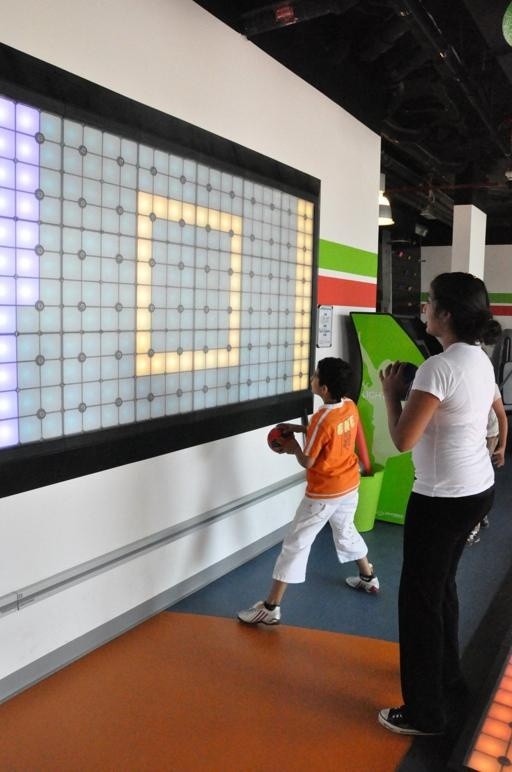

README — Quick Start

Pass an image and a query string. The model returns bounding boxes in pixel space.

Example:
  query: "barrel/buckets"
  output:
[353,463,385,532]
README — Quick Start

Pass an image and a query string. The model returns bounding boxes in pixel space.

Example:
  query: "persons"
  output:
[237,357,381,625]
[378,271,502,736]
[465,382,508,545]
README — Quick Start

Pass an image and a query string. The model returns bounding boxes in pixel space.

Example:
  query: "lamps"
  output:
[379,173,395,226]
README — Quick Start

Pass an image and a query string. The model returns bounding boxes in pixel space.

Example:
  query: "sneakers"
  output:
[379,707,446,735]
[345,576,379,593]
[237,602,280,625]
